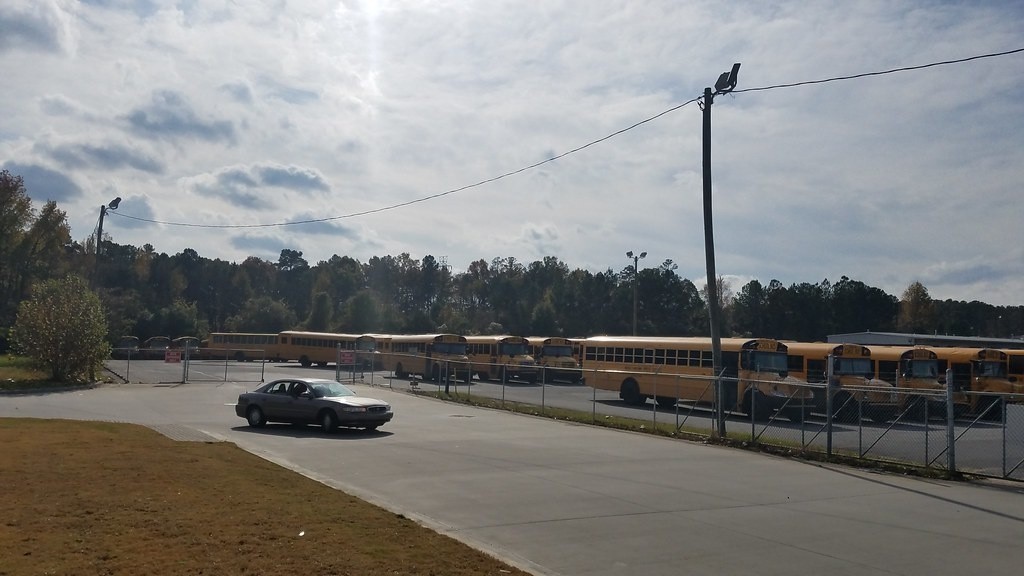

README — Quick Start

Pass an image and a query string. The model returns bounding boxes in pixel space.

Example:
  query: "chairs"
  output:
[279,384,286,393]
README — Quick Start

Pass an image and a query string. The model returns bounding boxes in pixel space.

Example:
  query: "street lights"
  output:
[626,251,647,336]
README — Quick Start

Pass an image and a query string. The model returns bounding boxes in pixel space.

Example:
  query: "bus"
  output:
[525,336,581,384]
[140,337,173,359]
[463,335,538,384]
[172,337,202,360]
[777,340,899,424]
[568,338,585,367]
[861,345,970,422]
[355,335,393,370]
[208,333,288,363]
[116,336,140,359]
[997,349,1024,386]
[581,334,817,423]
[277,331,360,368]
[388,334,473,383]
[926,347,1024,421]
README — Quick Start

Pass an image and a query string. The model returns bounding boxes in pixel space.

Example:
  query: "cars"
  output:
[235,378,393,435]
